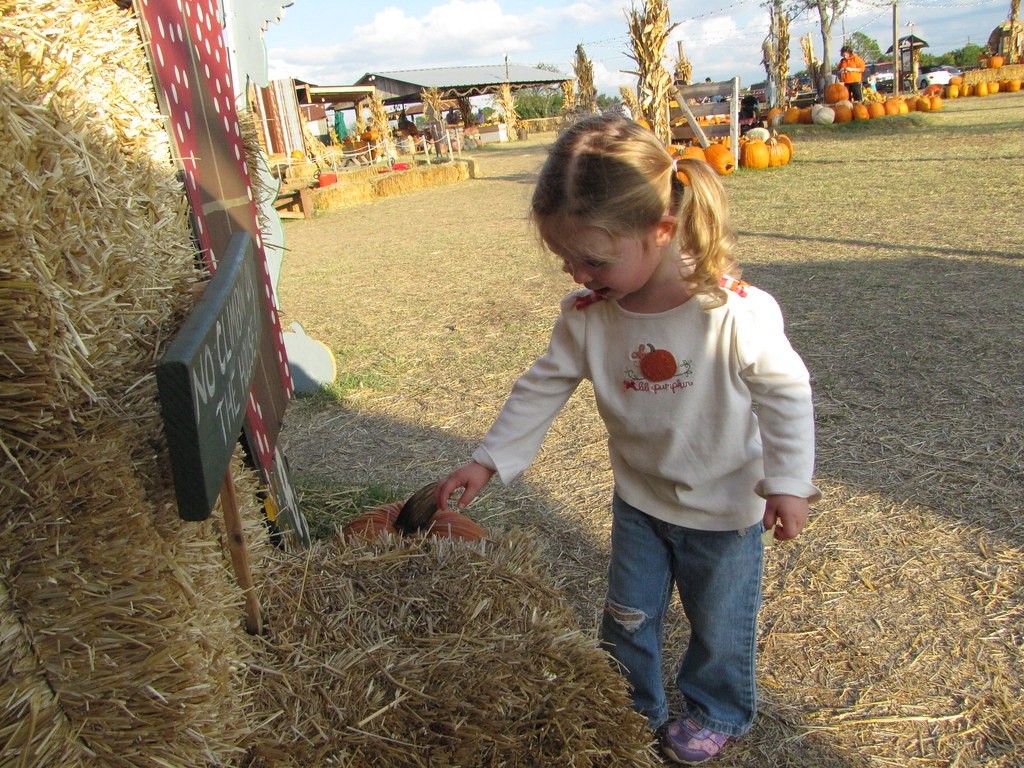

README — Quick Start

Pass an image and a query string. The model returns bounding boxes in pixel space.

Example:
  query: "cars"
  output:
[941,65,965,76]
[903,65,952,89]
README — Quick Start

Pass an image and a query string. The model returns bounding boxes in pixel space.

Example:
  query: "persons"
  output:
[704,77,723,103]
[867,70,878,92]
[432,117,825,768]
[834,45,866,102]
[620,102,634,117]
[787,78,797,89]
[475,107,485,125]
[674,72,689,104]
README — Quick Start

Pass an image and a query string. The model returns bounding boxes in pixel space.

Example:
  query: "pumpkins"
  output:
[987,52,1003,68]
[946,76,1020,101]
[339,482,487,544]
[361,130,381,159]
[633,114,793,186]
[292,149,305,160]
[768,78,945,128]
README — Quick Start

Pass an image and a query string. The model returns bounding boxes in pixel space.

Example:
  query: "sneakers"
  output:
[659,715,732,766]
